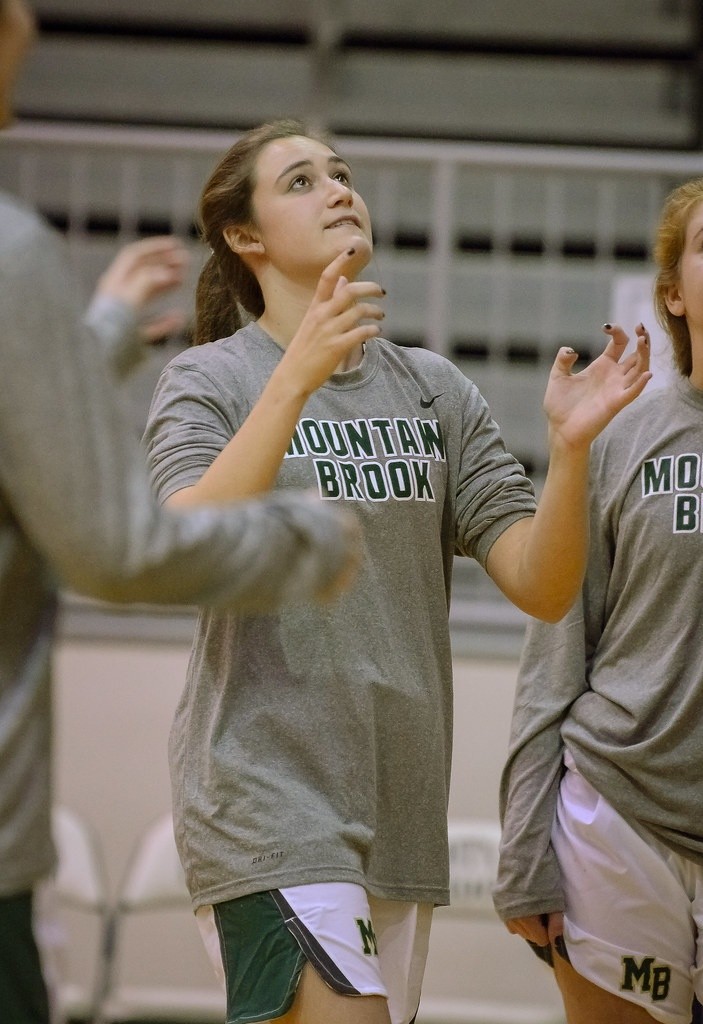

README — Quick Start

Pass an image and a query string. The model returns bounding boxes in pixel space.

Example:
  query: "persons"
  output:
[497,180,703,1024]
[135,120,652,1023]
[0,0,367,1023]
